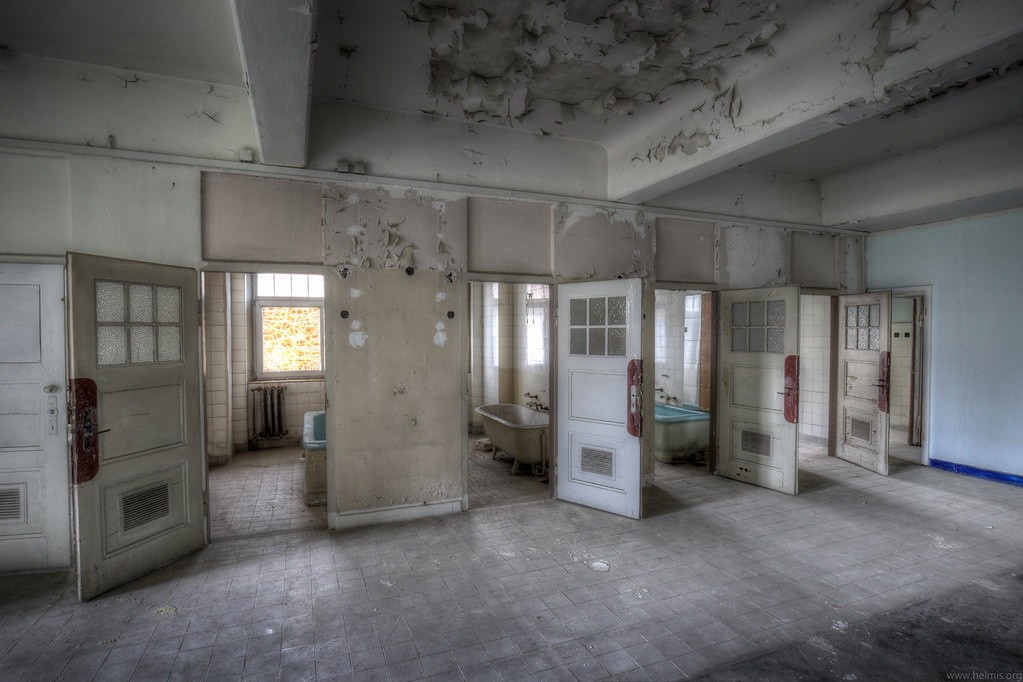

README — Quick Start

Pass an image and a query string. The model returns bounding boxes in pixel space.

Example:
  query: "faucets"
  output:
[534,403,548,410]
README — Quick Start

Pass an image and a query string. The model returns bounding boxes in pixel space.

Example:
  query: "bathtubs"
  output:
[302,409,328,505]
[476,403,551,466]
[654,400,711,464]
